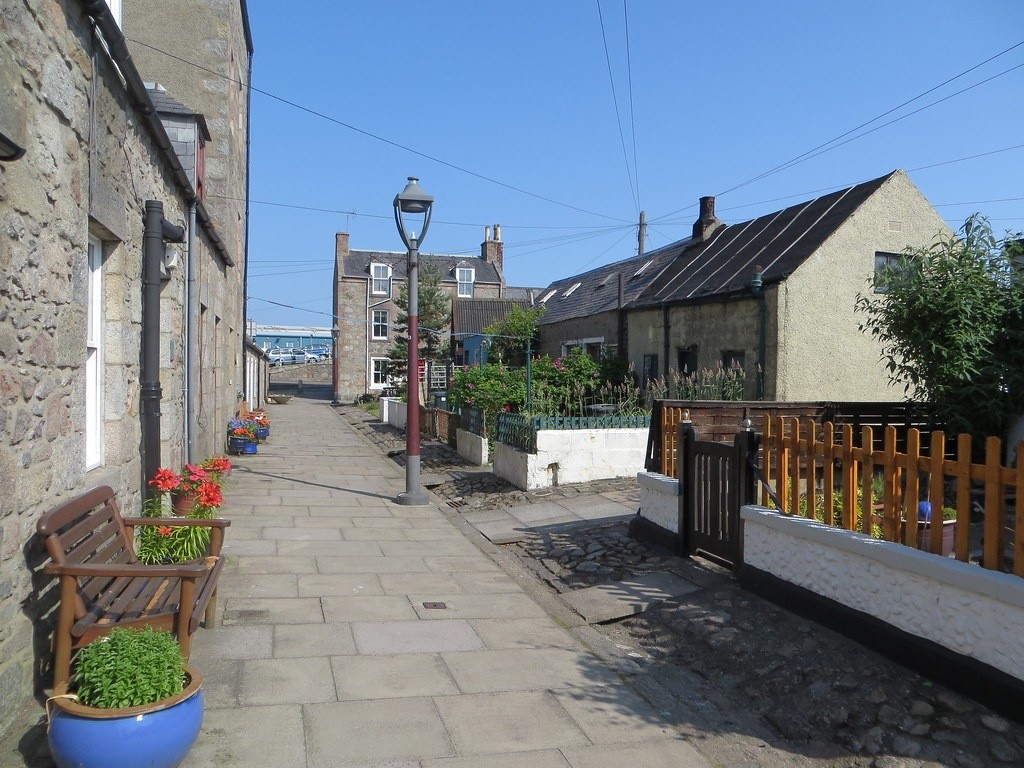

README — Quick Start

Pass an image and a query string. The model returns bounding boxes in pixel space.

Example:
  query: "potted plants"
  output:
[48,625,203,767]
[901,507,958,558]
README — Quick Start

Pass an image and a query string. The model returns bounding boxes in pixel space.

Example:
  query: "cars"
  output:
[265,347,320,366]
[297,344,329,361]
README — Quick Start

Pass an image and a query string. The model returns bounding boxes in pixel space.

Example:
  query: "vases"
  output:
[202,468,221,479]
[257,429,270,438]
[230,437,248,448]
[244,443,257,453]
[172,491,197,517]
[206,459,228,470]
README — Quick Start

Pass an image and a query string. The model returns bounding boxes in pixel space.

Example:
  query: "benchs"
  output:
[37,485,231,697]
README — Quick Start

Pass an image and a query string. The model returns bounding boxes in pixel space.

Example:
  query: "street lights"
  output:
[393,177,434,505]
[330,323,340,404]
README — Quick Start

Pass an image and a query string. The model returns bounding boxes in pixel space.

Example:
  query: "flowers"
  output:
[227,406,272,445]
[132,452,232,566]
